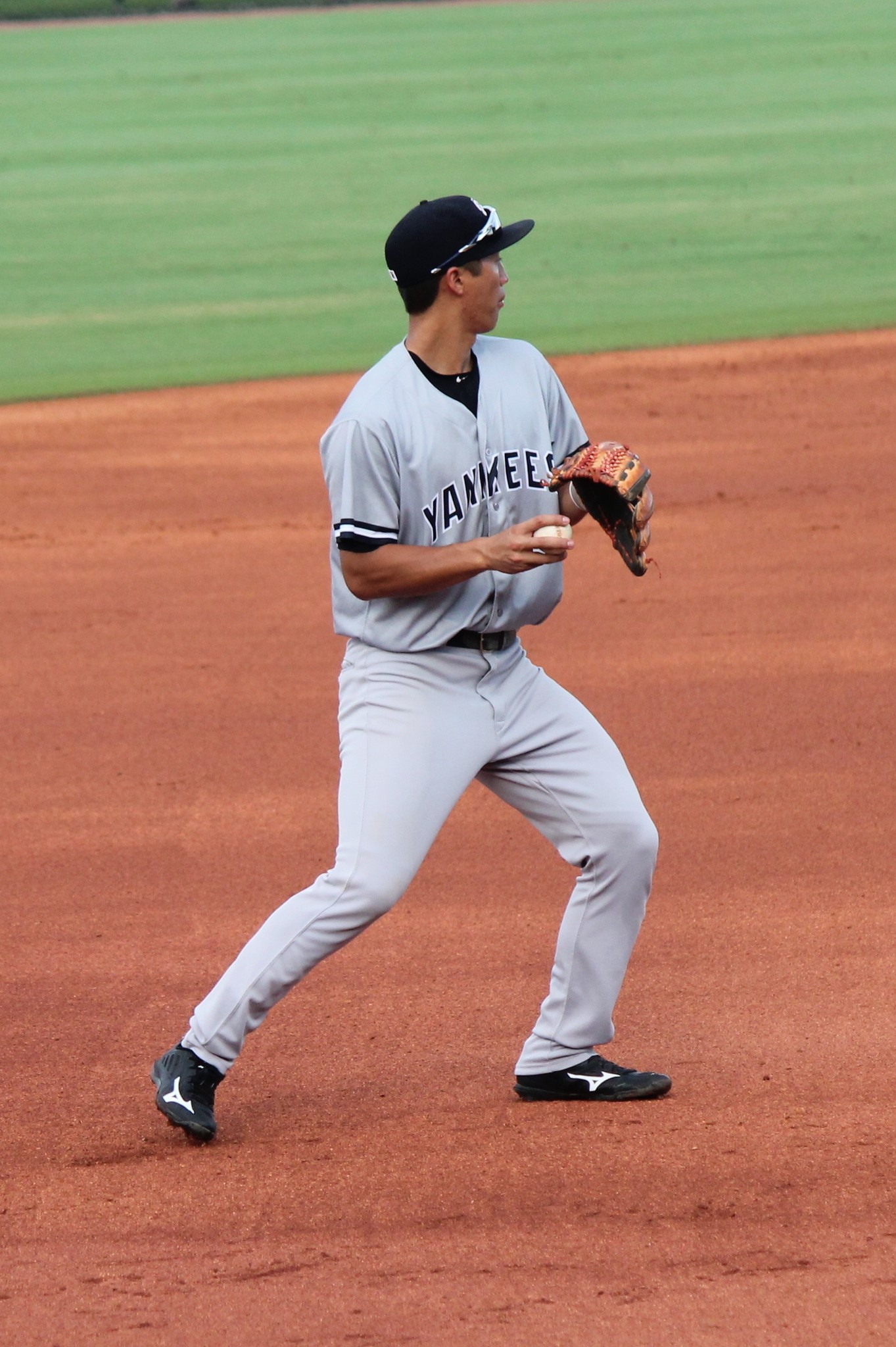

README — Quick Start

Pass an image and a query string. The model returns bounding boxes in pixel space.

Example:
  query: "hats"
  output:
[385,194,536,288]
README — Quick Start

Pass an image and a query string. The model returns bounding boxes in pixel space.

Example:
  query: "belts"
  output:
[447,628,517,652]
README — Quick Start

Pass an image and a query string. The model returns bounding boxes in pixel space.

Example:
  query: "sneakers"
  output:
[150,1036,226,1142]
[513,1054,674,1100]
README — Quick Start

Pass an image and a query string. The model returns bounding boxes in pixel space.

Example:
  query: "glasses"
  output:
[430,204,502,272]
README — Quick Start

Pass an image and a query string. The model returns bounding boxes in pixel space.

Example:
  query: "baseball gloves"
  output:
[540,440,664,580]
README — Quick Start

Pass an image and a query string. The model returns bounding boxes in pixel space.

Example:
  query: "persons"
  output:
[151,195,669,1136]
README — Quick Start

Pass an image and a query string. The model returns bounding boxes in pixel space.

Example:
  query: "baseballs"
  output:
[533,521,573,555]
